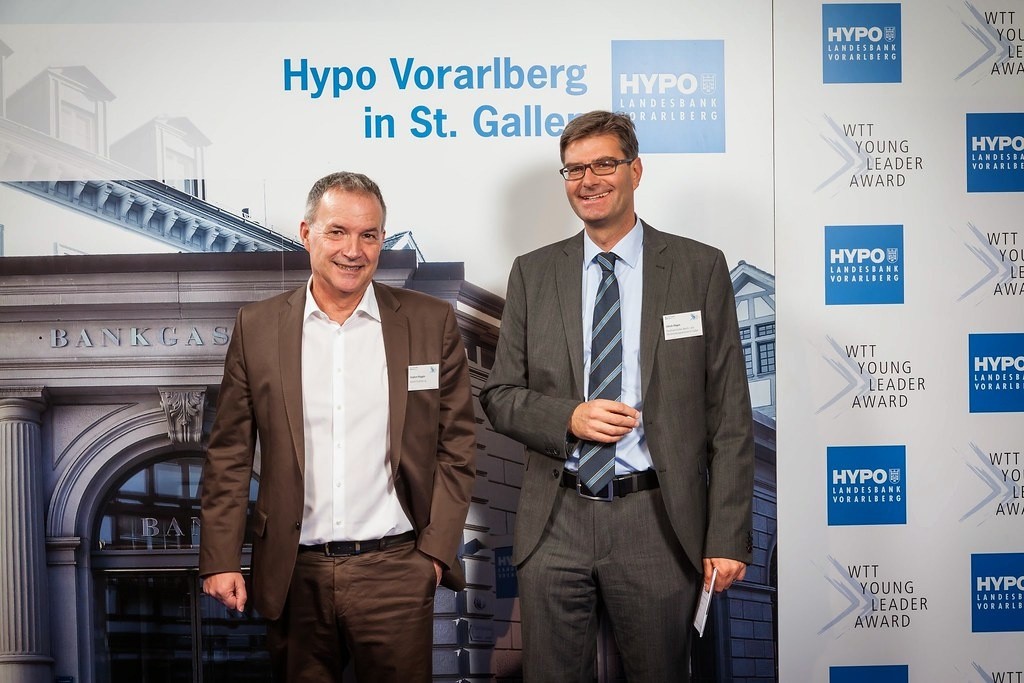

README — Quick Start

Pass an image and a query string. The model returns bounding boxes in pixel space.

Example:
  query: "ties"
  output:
[577,251,623,494]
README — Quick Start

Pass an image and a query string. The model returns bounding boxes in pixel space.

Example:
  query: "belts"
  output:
[561,469,660,502]
[297,529,415,557]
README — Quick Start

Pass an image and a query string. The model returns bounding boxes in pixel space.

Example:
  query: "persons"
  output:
[199,171,477,683]
[478,110,754,683]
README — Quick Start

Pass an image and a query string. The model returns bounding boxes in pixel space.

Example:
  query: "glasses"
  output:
[559,158,633,181]
